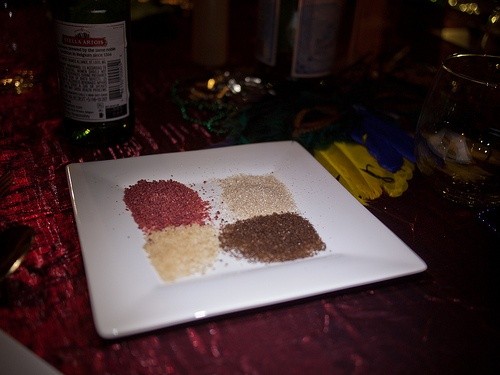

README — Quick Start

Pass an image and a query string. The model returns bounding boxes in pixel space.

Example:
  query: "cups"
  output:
[414,53,500,210]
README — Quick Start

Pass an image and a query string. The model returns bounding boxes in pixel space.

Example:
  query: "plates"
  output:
[66,140,428,341]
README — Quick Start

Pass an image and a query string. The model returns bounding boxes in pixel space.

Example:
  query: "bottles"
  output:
[191,0,255,68]
[54,0,134,148]
[251,1,346,105]
